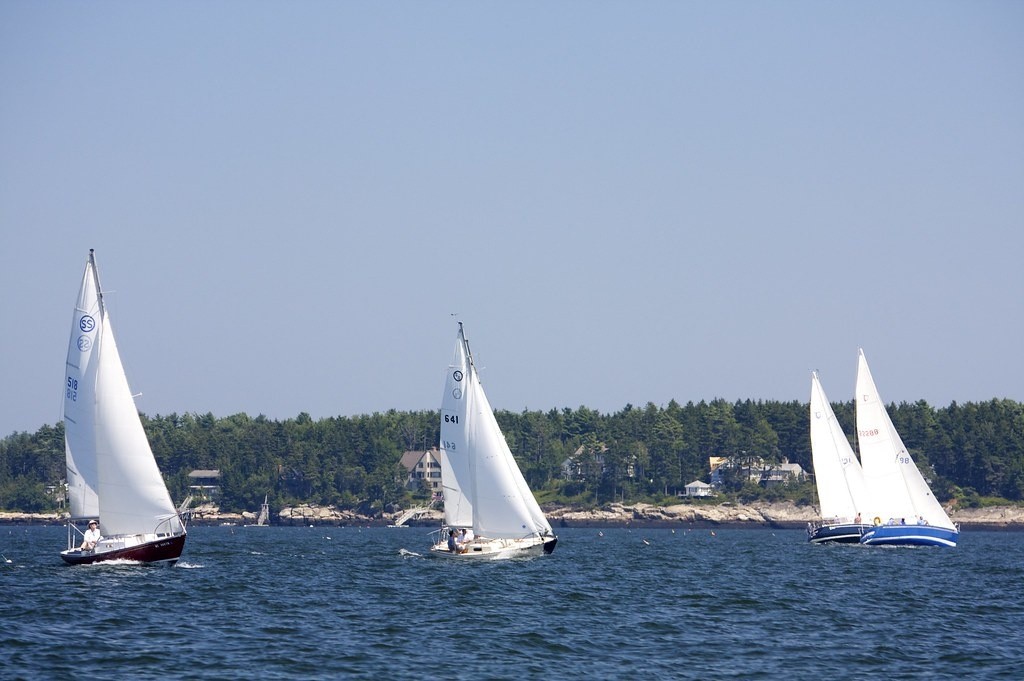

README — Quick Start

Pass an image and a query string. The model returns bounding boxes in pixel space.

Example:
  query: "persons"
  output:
[445,527,475,553]
[854,513,862,524]
[887,518,897,526]
[900,517,907,525]
[872,517,883,528]
[80,519,101,552]
[916,516,928,526]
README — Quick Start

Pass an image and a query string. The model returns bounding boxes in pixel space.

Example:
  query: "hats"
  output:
[449,530,453,537]
[87,520,98,529]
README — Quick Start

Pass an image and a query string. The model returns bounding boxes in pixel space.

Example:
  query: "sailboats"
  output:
[428,311,558,562]
[805,346,959,548]
[51,247,192,567]
